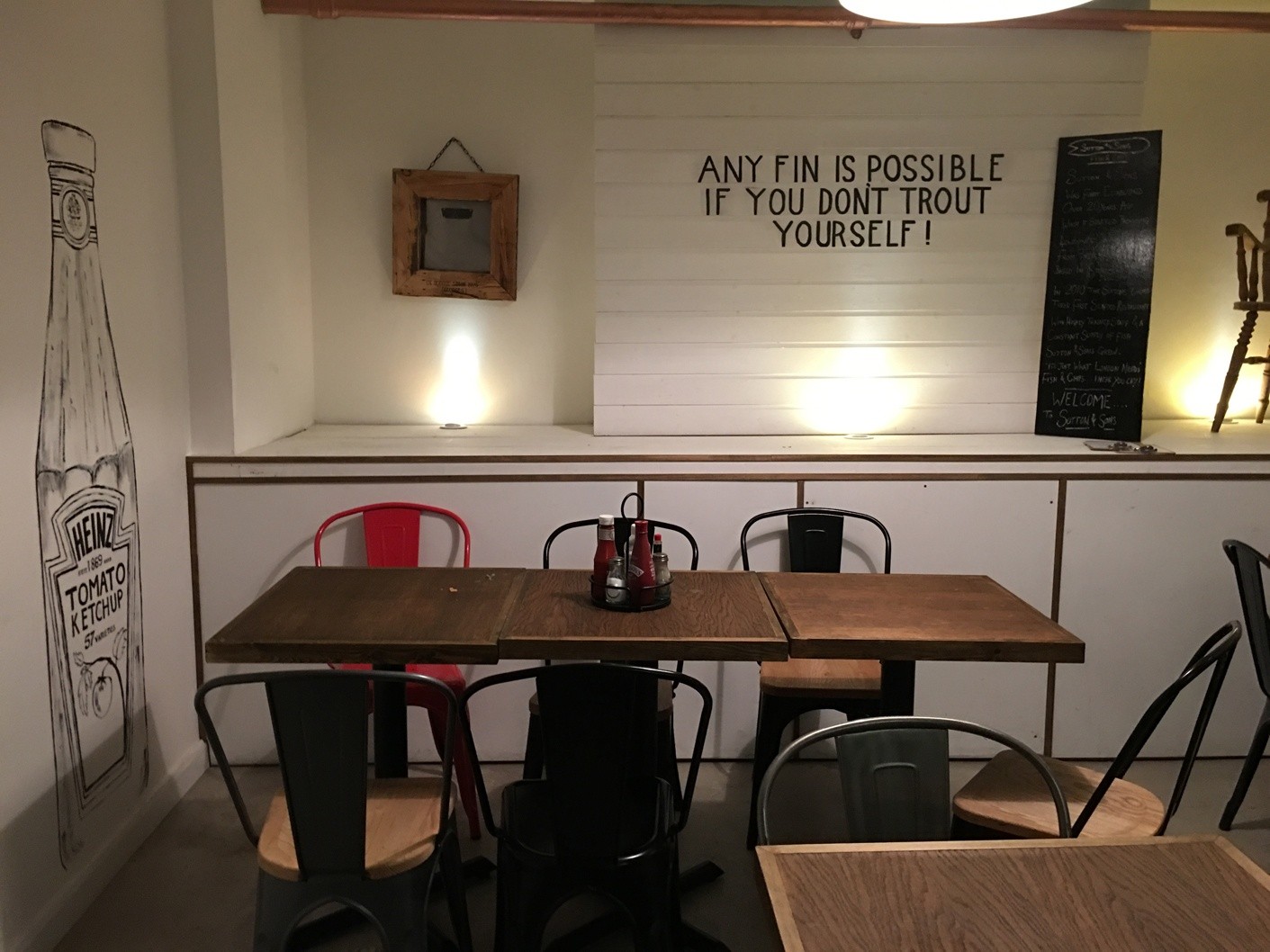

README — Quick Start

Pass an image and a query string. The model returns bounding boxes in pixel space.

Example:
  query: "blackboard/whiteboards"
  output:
[1034,130,1163,443]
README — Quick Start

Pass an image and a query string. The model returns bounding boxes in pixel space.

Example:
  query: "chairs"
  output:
[313,502,483,840]
[193,670,459,952]
[520,518,699,810]
[950,617,1243,841]
[457,660,711,952]
[756,714,1070,845]
[740,507,892,855]
[1219,537,1270,832]
[1211,190,1270,432]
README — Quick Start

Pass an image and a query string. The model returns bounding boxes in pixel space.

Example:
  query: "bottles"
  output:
[627,521,655,603]
[652,553,671,601]
[593,514,618,600]
[623,524,636,566]
[653,534,662,553]
[606,556,625,605]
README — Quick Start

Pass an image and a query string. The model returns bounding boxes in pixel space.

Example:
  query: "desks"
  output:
[757,836,1270,952]
[500,569,791,952]
[204,564,520,952]
[758,572,1085,717]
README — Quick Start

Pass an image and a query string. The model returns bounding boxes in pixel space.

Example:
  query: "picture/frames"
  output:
[390,166,520,301]
